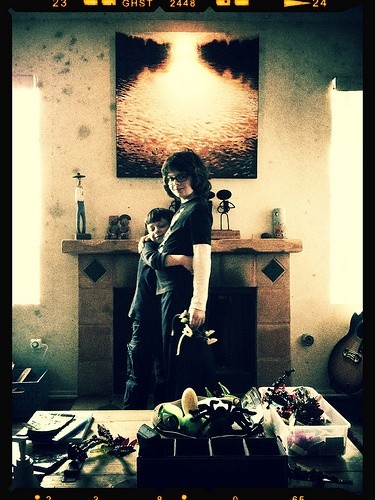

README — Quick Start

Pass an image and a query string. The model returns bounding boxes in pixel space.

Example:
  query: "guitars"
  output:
[327,310,363,393]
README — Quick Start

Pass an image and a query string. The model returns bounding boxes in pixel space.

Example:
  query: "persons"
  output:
[72,172,91,240]
[122,209,193,410]
[137,151,218,402]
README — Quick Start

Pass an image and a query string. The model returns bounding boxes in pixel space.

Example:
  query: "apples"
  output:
[181,413,204,437]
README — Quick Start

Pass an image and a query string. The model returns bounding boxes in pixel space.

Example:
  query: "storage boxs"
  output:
[259,386,351,458]
[153,396,263,439]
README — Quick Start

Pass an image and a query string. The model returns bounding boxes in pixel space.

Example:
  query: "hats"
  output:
[72,172,86,179]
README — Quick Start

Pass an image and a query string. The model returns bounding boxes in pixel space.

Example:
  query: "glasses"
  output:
[165,172,188,183]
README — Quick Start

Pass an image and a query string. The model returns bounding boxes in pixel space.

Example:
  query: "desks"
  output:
[12,410,362,488]
